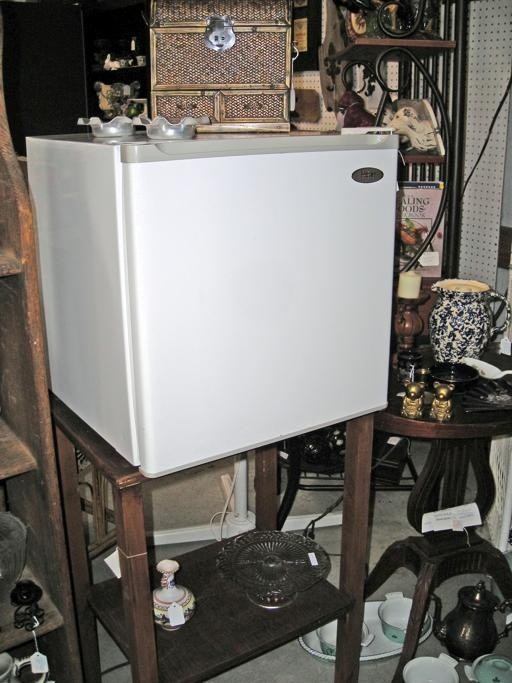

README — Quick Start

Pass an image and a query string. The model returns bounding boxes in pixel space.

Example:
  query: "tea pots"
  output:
[428,278,512,366]
[428,580,512,657]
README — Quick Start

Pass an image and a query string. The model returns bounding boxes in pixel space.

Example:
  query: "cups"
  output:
[311,591,512,683]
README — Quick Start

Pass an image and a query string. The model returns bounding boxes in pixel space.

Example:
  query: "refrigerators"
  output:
[23,128,396,480]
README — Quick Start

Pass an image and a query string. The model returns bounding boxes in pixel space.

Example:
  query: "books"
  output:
[371,93,446,156]
[397,179,444,278]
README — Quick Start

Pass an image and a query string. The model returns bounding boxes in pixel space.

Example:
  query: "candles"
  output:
[396,270,422,299]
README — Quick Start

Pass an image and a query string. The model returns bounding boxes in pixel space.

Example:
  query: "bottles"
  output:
[152,560,196,631]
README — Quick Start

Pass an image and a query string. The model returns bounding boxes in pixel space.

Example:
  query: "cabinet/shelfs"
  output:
[146,2,293,132]
[324,1,460,277]
[0,79,87,683]
[49,404,378,683]
[363,351,512,682]
